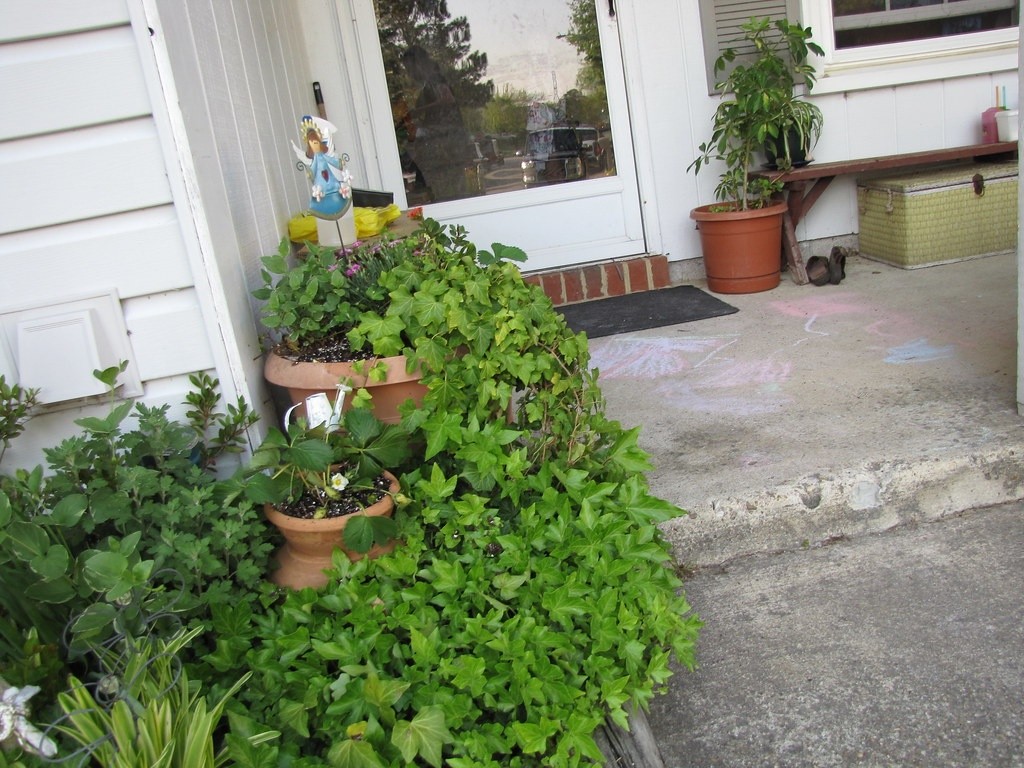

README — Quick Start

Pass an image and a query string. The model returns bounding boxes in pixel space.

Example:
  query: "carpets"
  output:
[553,285,740,339]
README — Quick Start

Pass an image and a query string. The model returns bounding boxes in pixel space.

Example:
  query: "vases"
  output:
[258,463,406,591]
[263,344,469,438]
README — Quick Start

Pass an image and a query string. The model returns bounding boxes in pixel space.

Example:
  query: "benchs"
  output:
[747,141,1019,285]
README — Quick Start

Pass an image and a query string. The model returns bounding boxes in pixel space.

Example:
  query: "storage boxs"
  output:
[995,110,1018,142]
[856,160,1018,270]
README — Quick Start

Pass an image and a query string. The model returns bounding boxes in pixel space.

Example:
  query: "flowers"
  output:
[250,207,470,360]
[201,406,406,553]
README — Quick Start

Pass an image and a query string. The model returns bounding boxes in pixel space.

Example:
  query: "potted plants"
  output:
[686,15,825,294]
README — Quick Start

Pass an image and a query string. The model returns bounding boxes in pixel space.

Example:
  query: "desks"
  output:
[296,209,422,259]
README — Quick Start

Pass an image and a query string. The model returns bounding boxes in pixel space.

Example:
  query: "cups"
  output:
[997,111,1019,143]
[982,108,1003,144]
